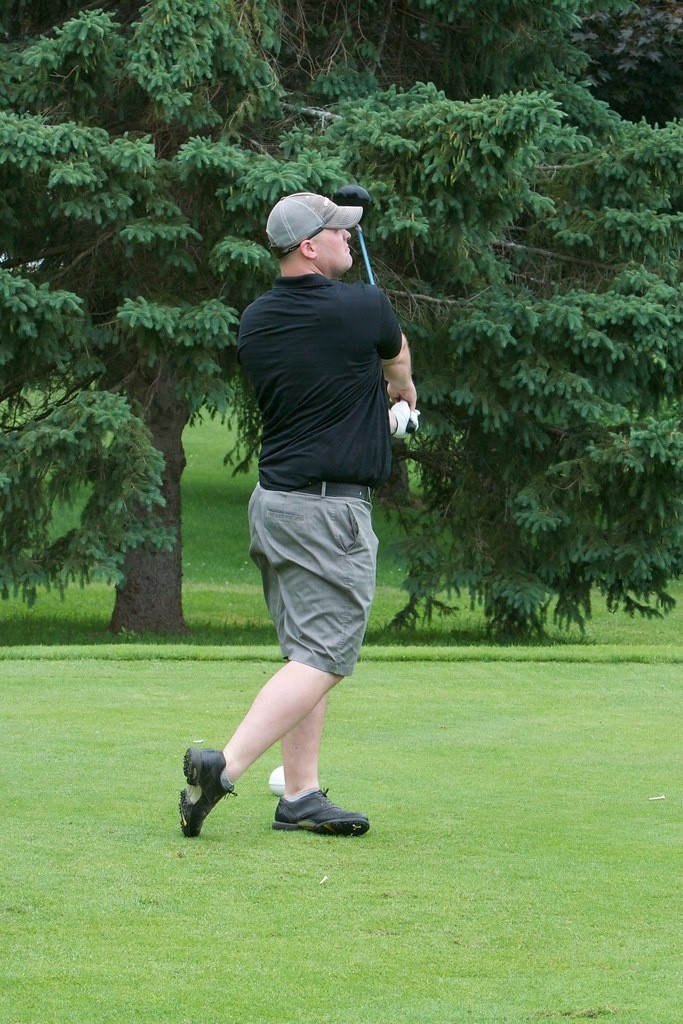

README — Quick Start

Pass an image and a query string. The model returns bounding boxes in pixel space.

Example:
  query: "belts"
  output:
[296,482,373,501]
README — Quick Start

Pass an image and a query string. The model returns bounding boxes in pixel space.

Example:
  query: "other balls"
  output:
[268,765,286,797]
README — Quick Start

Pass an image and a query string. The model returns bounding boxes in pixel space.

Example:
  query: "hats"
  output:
[266,192,363,248]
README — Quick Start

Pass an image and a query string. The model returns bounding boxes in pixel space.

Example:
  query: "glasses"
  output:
[268,228,323,259]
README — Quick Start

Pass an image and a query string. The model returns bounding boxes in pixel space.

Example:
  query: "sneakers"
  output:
[179,747,235,839]
[271,789,370,837]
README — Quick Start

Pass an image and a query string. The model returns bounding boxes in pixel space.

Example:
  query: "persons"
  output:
[181,193,417,838]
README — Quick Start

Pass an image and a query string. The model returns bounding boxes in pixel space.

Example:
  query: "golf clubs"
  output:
[333,183,418,435]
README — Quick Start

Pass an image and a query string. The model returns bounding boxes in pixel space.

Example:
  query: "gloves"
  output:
[390,399,421,439]
